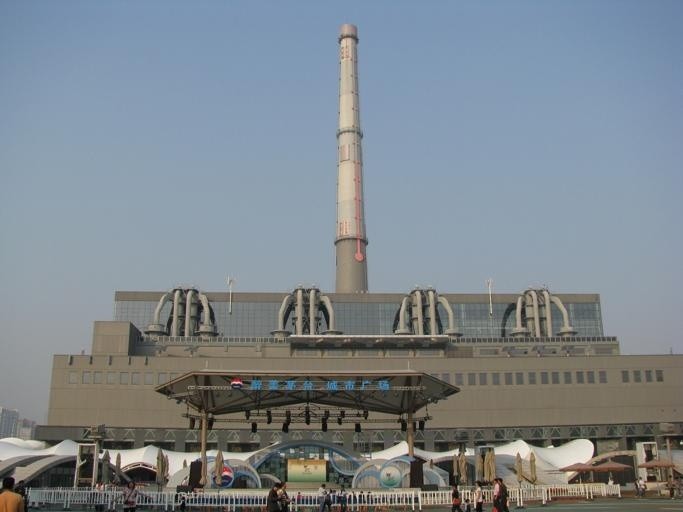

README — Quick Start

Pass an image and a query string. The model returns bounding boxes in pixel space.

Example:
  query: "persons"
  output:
[112,480,153,512]
[179,497,185,512]
[634,477,647,498]
[452,478,509,512]
[0,476,25,512]
[88,481,104,504]
[668,476,683,499]
[608,478,615,485]
[266,482,373,512]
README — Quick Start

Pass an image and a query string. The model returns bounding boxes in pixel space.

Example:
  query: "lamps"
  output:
[244,408,369,434]
[399,419,426,432]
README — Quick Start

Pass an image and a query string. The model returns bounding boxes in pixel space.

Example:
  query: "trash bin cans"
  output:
[661,484,677,497]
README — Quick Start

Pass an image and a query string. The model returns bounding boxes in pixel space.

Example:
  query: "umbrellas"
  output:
[530,453,537,486]
[638,459,675,493]
[453,449,497,485]
[156,446,169,493]
[199,448,207,492]
[594,461,633,478]
[516,453,523,487]
[183,460,187,486]
[102,450,121,484]
[559,463,594,484]
[430,459,433,469]
[215,449,224,494]
